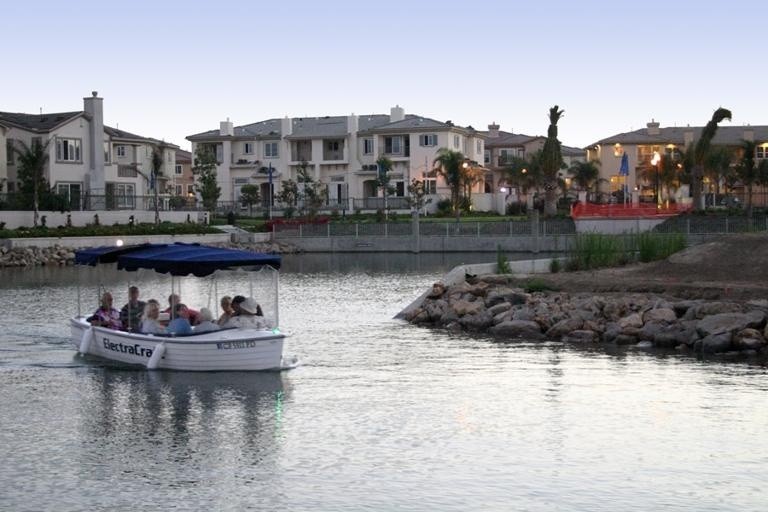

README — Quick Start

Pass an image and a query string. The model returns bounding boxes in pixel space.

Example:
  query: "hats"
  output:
[239,297,258,314]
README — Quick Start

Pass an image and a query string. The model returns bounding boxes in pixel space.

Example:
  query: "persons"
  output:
[91,287,264,337]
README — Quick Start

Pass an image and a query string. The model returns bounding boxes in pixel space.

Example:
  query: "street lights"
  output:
[649,152,661,204]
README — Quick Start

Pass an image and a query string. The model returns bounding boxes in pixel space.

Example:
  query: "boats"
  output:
[65,239,289,374]
